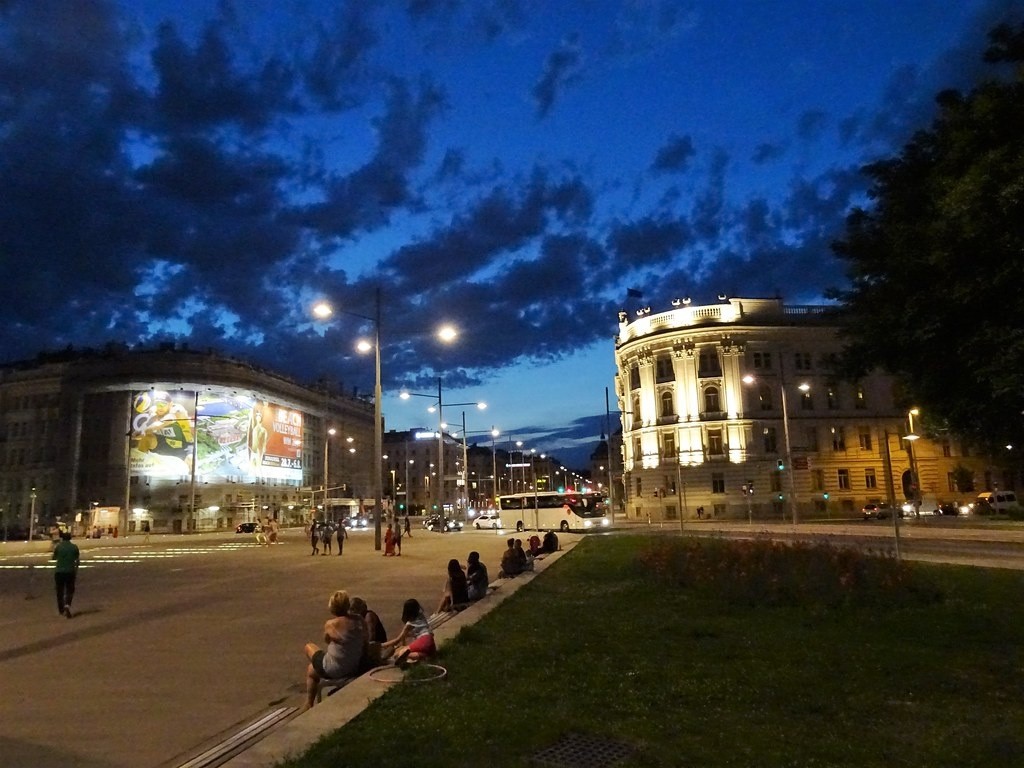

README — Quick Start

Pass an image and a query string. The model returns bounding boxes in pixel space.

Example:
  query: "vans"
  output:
[901,498,938,516]
[978,491,1017,514]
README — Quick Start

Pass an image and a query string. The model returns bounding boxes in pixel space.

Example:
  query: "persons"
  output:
[430,559,469,616]
[401,517,412,537]
[50,523,119,551]
[304,518,348,556]
[466,530,559,603]
[143,522,151,543]
[52,533,80,619]
[254,517,280,546]
[349,597,388,643]
[383,524,396,556]
[380,599,436,665]
[304,590,368,709]
[392,517,401,556]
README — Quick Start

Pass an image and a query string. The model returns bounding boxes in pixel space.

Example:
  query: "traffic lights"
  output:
[777,459,783,470]
[779,495,783,499]
[824,493,828,499]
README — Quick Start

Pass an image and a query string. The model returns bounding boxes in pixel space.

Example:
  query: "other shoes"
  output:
[400,660,420,671]
[60,611,64,614]
[63,607,72,620]
[394,647,410,667]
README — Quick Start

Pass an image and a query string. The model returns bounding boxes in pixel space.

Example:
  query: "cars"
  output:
[237,523,257,533]
[351,514,374,528]
[458,507,500,529]
[959,500,992,515]
[422,513,463,532]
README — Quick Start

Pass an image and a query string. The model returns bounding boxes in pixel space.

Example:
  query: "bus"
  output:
[498,490,606,532]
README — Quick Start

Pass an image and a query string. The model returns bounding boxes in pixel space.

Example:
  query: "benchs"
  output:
[178,704,305,768]
[327,549,554,693]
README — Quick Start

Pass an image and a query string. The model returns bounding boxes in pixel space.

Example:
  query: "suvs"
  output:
[863,504,893,520]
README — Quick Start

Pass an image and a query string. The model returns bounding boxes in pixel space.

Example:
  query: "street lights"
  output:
[315,427,355,524]
[884,407,921,560]
[491,426,588,501]
[400,381,486,531]
[744,349,810,526]
[89,501,99,538]
[314,282,458,551]
[161,391,238,535]
